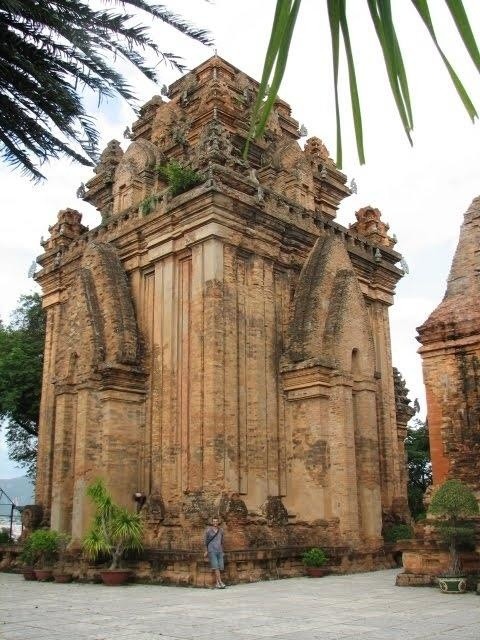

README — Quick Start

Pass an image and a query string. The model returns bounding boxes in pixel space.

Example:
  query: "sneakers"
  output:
[216,582,226,589]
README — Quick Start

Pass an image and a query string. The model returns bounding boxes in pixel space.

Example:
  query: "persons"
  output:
[202,517,228,589]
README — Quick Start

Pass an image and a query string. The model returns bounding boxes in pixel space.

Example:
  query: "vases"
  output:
[99,569,134,586]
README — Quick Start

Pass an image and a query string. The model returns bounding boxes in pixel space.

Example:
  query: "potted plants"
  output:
[81,529,109,584]
[427,480,478,594]
[48,532,72,583]
[30,530,53,581]
[19,537,36,581]
[302,548,329,577]
